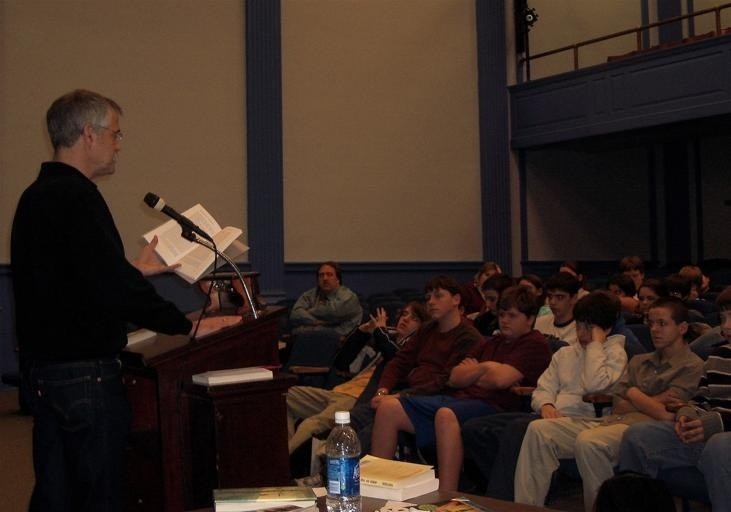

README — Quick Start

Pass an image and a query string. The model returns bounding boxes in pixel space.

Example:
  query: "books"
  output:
[376,493,494,511]
[359,453,436,488]
[192,365,274,385]
[143,202,252,287]
[211,485,319,512]
[358,479,441,501]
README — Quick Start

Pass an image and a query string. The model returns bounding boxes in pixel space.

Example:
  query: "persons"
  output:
[620,255,645,288]
[7,87,241,512]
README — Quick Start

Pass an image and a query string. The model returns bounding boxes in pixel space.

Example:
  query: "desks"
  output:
[214,476,545,511]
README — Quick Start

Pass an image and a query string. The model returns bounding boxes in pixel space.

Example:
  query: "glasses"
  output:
[92,122,125,143]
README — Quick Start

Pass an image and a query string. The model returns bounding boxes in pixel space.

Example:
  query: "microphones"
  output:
[143,192,213,241]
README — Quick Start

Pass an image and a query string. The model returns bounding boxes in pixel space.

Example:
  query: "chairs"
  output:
[276,269,731,512]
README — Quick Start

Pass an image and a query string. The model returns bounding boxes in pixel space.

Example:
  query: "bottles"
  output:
[325,410,362,512]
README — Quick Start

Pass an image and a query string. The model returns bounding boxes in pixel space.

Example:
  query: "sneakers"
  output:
[291,470,326,488]
